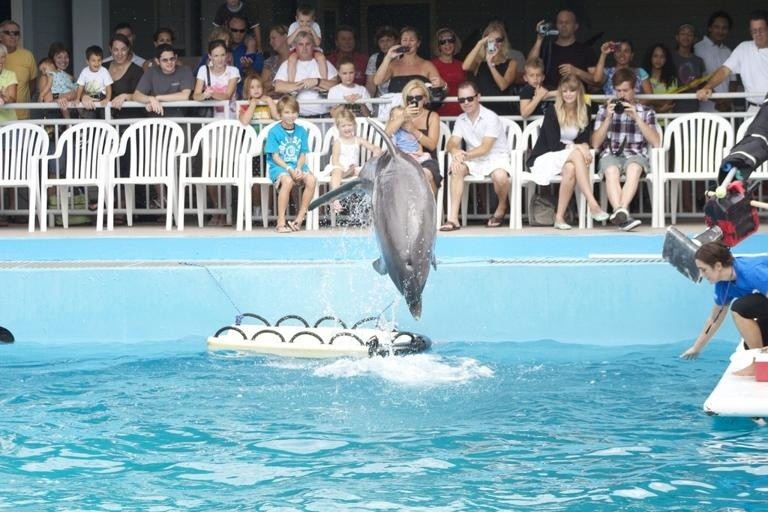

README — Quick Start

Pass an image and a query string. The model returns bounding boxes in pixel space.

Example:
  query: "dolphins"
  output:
[306,118,437,319]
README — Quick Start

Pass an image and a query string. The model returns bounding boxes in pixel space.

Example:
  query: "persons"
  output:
[679,234,768,377]
[1,1,766,232]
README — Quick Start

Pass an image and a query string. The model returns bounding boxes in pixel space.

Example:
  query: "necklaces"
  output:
[705,266,734,333]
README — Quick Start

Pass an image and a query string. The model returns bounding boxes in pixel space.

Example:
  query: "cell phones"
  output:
[411,99,418,115]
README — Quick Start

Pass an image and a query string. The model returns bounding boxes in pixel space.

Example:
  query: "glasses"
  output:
[457,94,478,103]
[0,29,22,37]
[438,38,455,44]
[406,96,424,102]
[495,38,504,43]
[231,28,246,33]
[161,57,177,63]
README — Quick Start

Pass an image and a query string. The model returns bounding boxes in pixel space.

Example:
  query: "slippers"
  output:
[486,209,507,227]
[439,222,461,231]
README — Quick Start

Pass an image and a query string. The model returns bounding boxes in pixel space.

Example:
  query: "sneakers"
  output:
[276,221,301,233]
[553,220,572,230]
[589,208,642,231]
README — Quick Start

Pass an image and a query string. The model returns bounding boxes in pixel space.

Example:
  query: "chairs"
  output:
[516,116,588,231]
[447,116,522,232]
[434,119,453,232]
[658,112,736,230]
[106,117,185,232]
[243,118,323,233]
[736,116,768,182]
[176,119,259,232]
[313,115,386,233]
[585,121,664,230]
[38,121,120,233]
[0,122,50,233]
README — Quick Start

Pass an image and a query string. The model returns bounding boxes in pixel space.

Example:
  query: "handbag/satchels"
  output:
[184,90,213,118]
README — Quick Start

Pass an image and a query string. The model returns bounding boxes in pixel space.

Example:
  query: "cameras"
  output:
[609,43,621,52]
[488,41,496,53]
[396,47,410,52]
[538,21,559,37]
[610,98,629,114]
